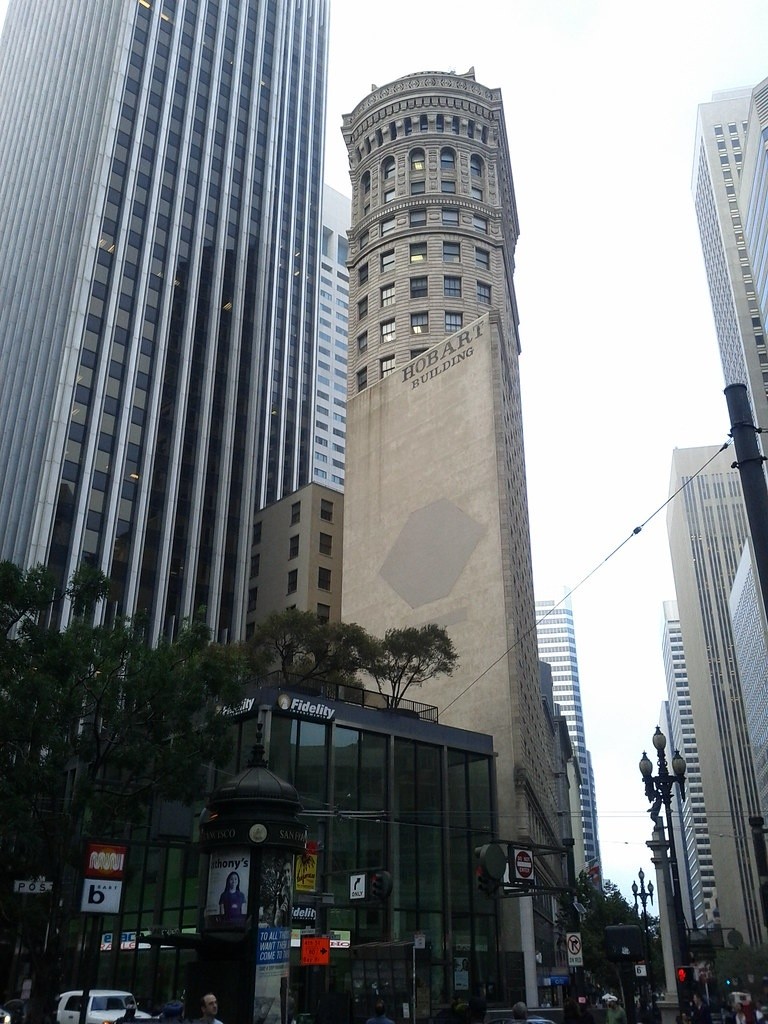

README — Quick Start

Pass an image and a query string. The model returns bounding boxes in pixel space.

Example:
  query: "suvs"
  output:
[55,988,152,1023]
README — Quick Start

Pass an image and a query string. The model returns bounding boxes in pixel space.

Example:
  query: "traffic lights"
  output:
[476,843,507,888]
[369,870,393,899]
[676,966,694,986]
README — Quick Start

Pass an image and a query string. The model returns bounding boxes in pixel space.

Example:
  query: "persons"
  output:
[460,959,469,971]
[605,995,663,1024]
[273,860,292,926]
[114,1004,136,1024]
[677,991,713,1024]
[512,1002,532,1024]
[733,1000,768,1024]
[445,996,487,1024]
[192,992,223,1024]
[563,997,594,1024]
[366,1003,395,1024]
[218,872,246,914]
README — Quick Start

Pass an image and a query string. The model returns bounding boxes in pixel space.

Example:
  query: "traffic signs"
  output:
[349,873,365,899]
[299,937,330,966]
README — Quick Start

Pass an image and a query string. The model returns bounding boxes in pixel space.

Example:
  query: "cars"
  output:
[488,1016,555,1024]
[0,998,28,1024]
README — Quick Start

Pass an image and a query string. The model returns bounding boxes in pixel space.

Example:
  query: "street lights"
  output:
[630,868,659,1010]
[640,725,693,966]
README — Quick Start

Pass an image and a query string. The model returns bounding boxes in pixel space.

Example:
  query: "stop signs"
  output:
[514,849,535,880]
